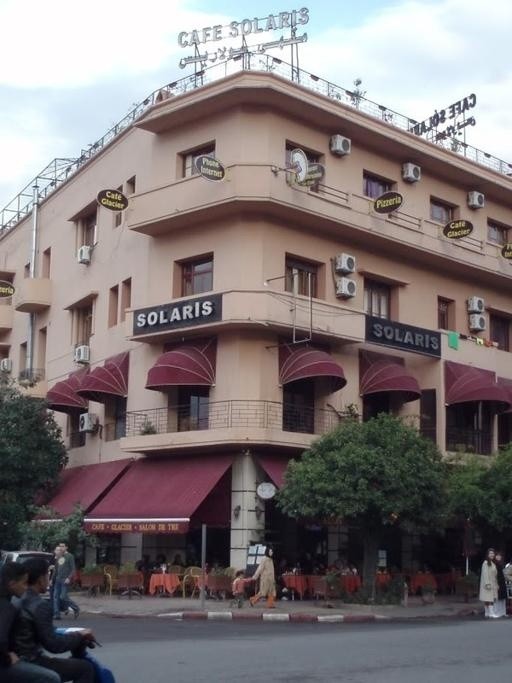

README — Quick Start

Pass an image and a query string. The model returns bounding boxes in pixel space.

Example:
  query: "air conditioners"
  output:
[468,296,487,333]
[330,134,352,157]
[334,252,357,300]
[77,245,92,266]
[0,358,12,374]
[78,413,97,434]
[402,162,422,183]
[467,190,486,210]
[75,346,90,364]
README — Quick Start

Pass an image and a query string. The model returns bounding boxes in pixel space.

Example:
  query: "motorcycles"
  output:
[55,626,115,683]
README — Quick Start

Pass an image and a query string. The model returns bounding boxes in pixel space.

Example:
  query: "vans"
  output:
[0,550,54,600]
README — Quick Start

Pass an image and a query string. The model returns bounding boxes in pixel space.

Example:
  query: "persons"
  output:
[136,551,150,594]
[479,547,500,619]
[444,560,458,596]
[47,545,69,616]
[0,560,61,683]
[11,557,98,683]
[49,541,82,620]
[275,551,360,601]
[493,551,509,616]
[248,546,277,609]
[154,555,167,567]
[170,554,184,567]
[229,571,255,609]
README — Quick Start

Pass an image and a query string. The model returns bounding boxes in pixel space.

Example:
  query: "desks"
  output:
[280,570,462,602]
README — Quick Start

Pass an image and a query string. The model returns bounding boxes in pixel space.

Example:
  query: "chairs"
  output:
[103,563,235,597]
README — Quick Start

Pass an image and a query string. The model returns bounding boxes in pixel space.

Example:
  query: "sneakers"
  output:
[52,604,80,619]
[250,597,254,608]
[266,605,276,608]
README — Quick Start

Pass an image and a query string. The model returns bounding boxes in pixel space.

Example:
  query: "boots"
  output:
[484,605,499,618]
